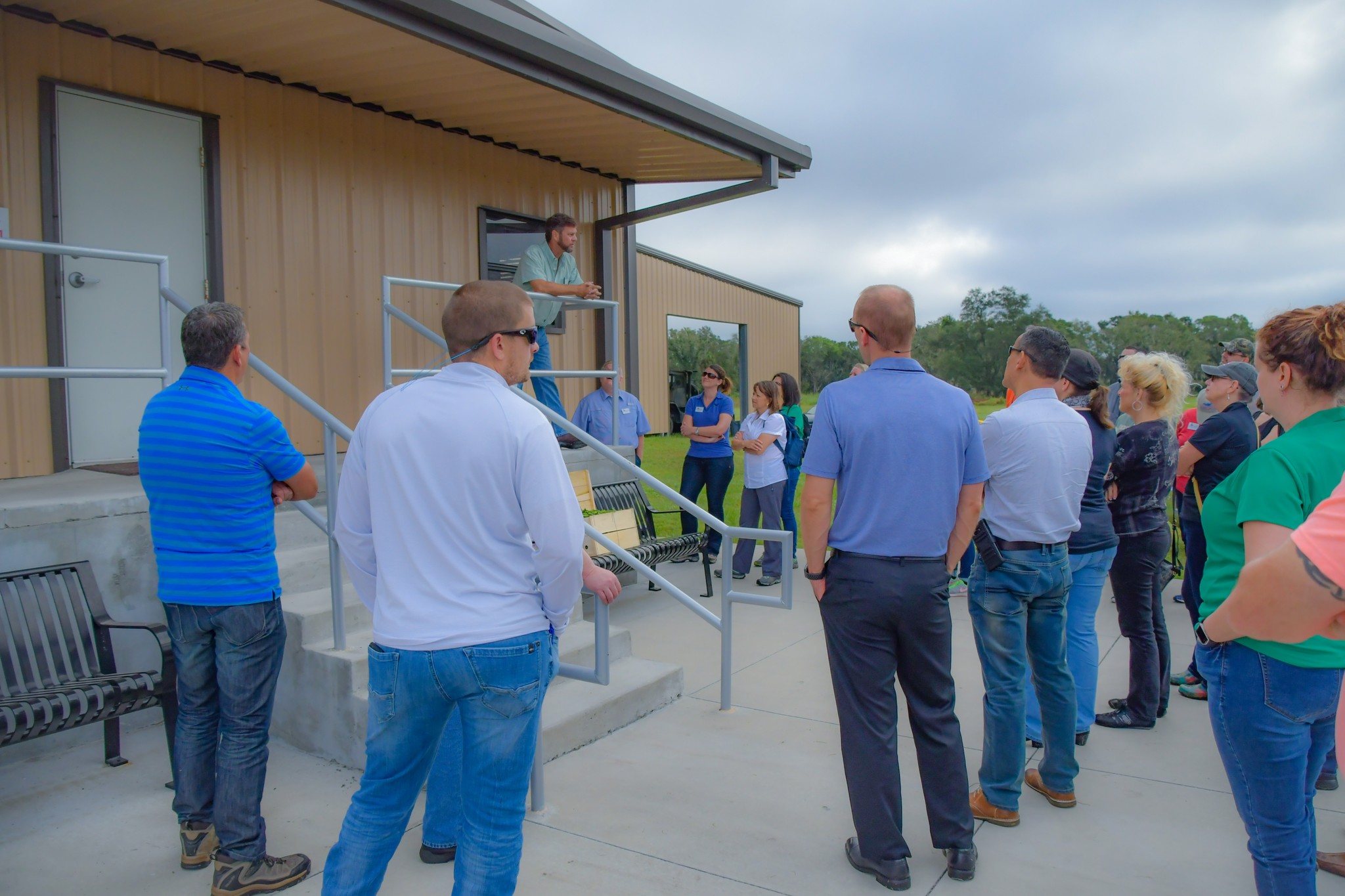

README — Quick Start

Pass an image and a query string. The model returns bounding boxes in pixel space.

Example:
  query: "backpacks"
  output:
[787,404,812,458]
[763,411,805,502]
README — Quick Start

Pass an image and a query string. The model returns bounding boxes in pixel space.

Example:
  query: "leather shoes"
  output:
[845,836,912,890]
[942,841,979,882]
[1021,768,1078,807]
[969,788,1020,829]
[1314,774,1339,790]
[1315,852,1345,876]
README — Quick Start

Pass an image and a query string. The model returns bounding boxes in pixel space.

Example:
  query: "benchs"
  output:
[0,560,183,790]
[588,478,715,598]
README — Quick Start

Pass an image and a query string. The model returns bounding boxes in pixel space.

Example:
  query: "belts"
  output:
[830,547,946,564]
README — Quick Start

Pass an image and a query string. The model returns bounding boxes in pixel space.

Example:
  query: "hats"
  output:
[1062,348,1100,391]
[1217,338,1255,361]
[1201,362,1259,396]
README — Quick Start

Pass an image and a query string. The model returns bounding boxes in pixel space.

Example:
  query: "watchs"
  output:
[1195,618,1223,648]
[804,562,826,580]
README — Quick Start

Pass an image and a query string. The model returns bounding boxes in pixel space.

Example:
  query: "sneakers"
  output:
[176,819,222,871]
[792,557,799,569]
[715,568,746,580]
[208,850,311,896]
[421,844,457,863]
[757,576,781,586]
[1096,705,1156,731]
[1109,698,1168,718]
[669,555,699,562]
[702,554,717,563]
[754,551,765,566]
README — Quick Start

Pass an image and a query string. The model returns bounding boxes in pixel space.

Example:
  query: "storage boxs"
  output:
[566,469,642,559]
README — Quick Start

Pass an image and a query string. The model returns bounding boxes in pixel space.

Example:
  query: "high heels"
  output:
[1027,738,1044,748]
[1075,731,1089,745]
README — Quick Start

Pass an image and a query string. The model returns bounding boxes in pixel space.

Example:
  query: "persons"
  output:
[138,302,318,896]
[753,372,804,569]
[714,380,787,586]
[800,283,992,892]
[417,544,622,864]
[948,539,976,597]
[510,213,603,450]
[570,360,653,468]
[320,276,588,896]
[1109,412,1175,605]
[1192,300,1345,896]
[1023,348,1120,749]
[1251,396,1285,447]
[669,364,735,564]
[968,326,1094,828]
[1172,389,1220,604]
[1235,469,1345,877]
[1105,344,1152,425]
[1218,338,1263,416]
[848,363,869,377]
[1094,351,1193,730]
[1168,362,1261,701]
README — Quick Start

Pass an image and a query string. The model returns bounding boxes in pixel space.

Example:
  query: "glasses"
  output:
[1223,347,1247,358]
[473,326,537,351]
[1211,375,1239,389]
[849,318,921,353]
[701,371,720,380]
[1119,355,1127,360]
[1008,346,1038,363]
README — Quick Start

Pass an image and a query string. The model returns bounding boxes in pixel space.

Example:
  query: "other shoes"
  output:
[558,433,588,450]
[1169,669,1202,686]
[1178,682,1208,701]
